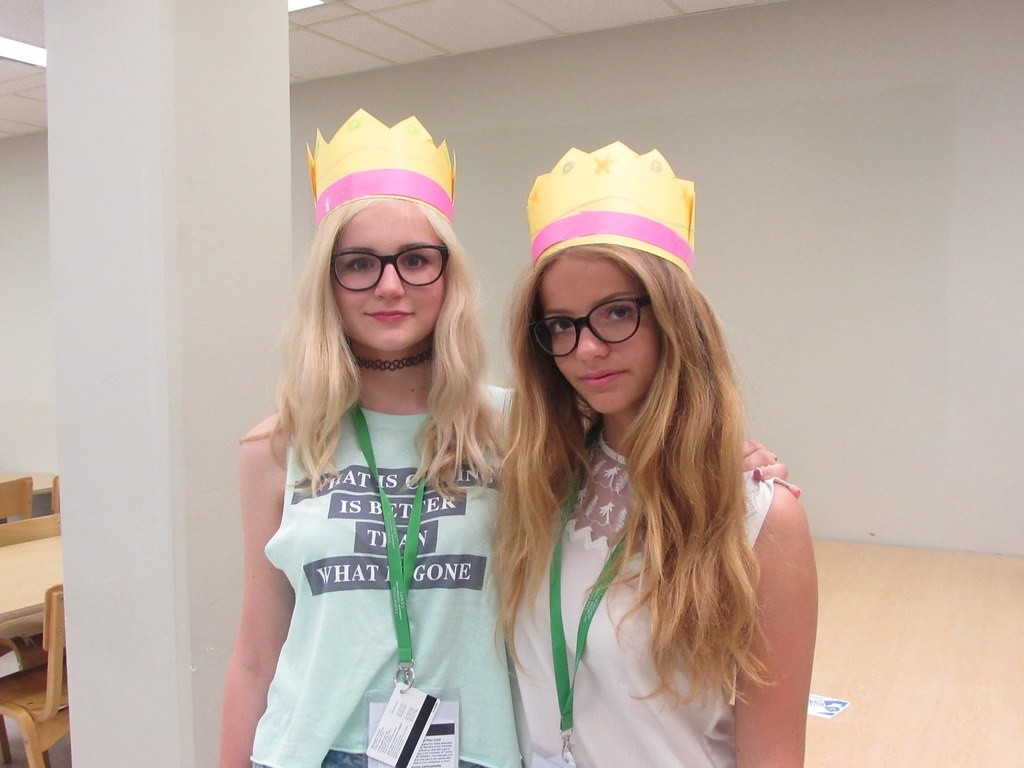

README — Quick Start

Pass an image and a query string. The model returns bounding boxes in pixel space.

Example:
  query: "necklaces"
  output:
[355,349,430,371]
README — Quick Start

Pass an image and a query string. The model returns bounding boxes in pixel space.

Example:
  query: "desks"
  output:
[0,535,63,640]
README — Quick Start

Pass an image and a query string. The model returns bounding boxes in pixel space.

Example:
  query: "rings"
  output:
[770,453,778,463]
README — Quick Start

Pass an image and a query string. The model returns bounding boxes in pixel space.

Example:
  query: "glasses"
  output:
[332,245,448,292]
[533,294,651,357]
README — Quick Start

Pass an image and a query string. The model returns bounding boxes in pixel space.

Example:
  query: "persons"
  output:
[496,141,819,768]
[219,109,803,768]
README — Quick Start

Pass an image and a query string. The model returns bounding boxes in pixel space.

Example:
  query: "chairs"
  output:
[0,476,72,768]
